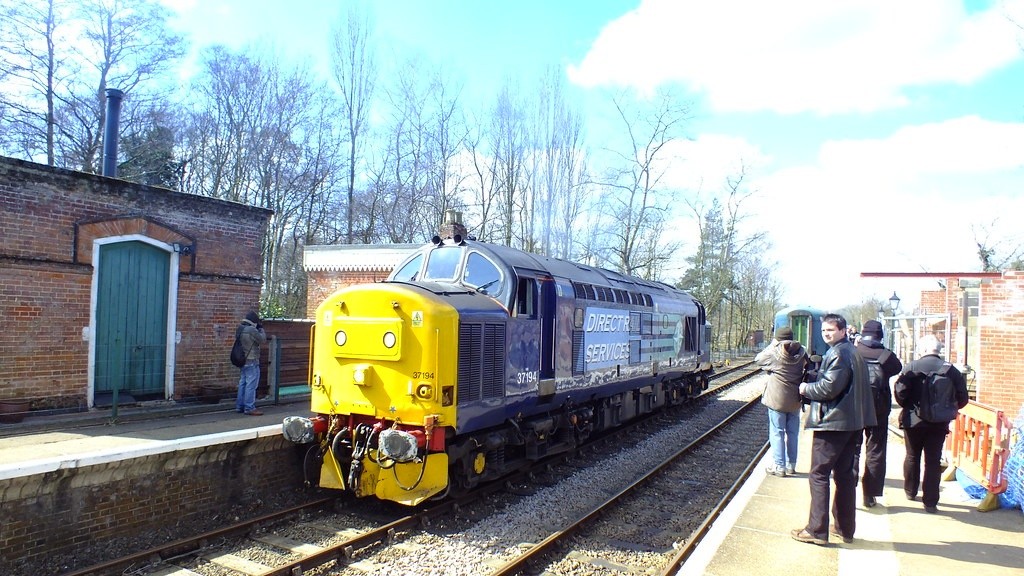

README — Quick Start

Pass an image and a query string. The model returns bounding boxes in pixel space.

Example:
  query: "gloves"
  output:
[256,325,262,330]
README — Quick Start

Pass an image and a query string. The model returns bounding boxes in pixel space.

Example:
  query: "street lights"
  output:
[889,291,900,354]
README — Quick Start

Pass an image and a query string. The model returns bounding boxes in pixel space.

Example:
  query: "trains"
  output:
[283,209,714,507]
[774,306,829,384]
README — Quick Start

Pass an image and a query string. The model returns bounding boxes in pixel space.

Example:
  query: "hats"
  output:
[862,321,883,340]
[776,326,794,341]
[246,311,259,323]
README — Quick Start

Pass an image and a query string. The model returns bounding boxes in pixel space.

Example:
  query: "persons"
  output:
[894,334,969,511]
[791,314,877,545]
[857,320,903,506]
[236,311,266,415]
[847,324,861,346]
[755,327,808,476]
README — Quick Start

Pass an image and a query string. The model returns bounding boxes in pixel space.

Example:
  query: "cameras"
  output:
[257,320,262,328]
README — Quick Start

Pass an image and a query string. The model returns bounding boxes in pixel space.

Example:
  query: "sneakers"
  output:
[830,524,852,543]
[766,466,786,477]
[791,528,827,544]
[786,468,795,474]
[245,408,263,415]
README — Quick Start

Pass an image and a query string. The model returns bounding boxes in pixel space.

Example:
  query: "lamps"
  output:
[173,243,195,256]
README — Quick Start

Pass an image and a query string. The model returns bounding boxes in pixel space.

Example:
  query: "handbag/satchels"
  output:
[799,350,823,412]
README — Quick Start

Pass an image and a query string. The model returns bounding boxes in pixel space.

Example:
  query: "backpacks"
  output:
[909,361,958,421]
[230,325,254,368]
[866,348,892,408]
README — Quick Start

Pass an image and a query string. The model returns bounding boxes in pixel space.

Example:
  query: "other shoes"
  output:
[864,495,876,507]
[906,494,915,500]
[924,504,937,513]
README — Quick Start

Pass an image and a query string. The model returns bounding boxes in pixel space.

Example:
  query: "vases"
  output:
[0,399,31,424]
[202,383,225,404]
